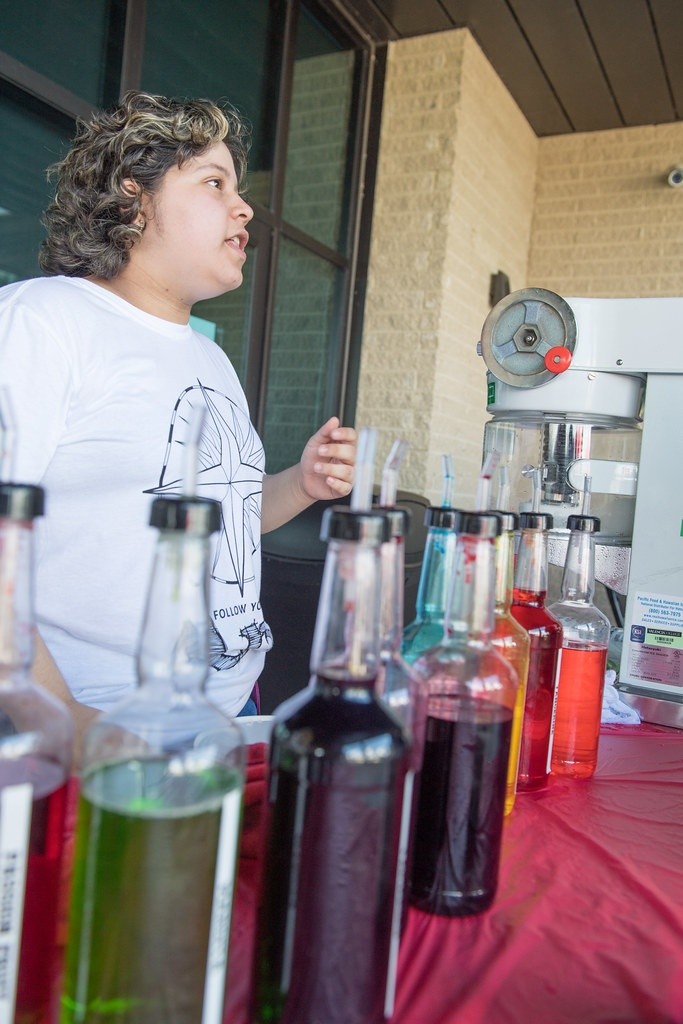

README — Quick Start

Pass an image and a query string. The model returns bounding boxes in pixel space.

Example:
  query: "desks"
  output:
[17,691,683,1024]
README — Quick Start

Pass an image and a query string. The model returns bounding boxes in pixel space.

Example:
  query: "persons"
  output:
[0,90,356,809]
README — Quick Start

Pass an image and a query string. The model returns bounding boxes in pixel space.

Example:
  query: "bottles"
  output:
[546,515,610,781]
[510,512,563,792]
[247,507,529,1024]
[1,480,77,1024]
[61,495,247,1023]
